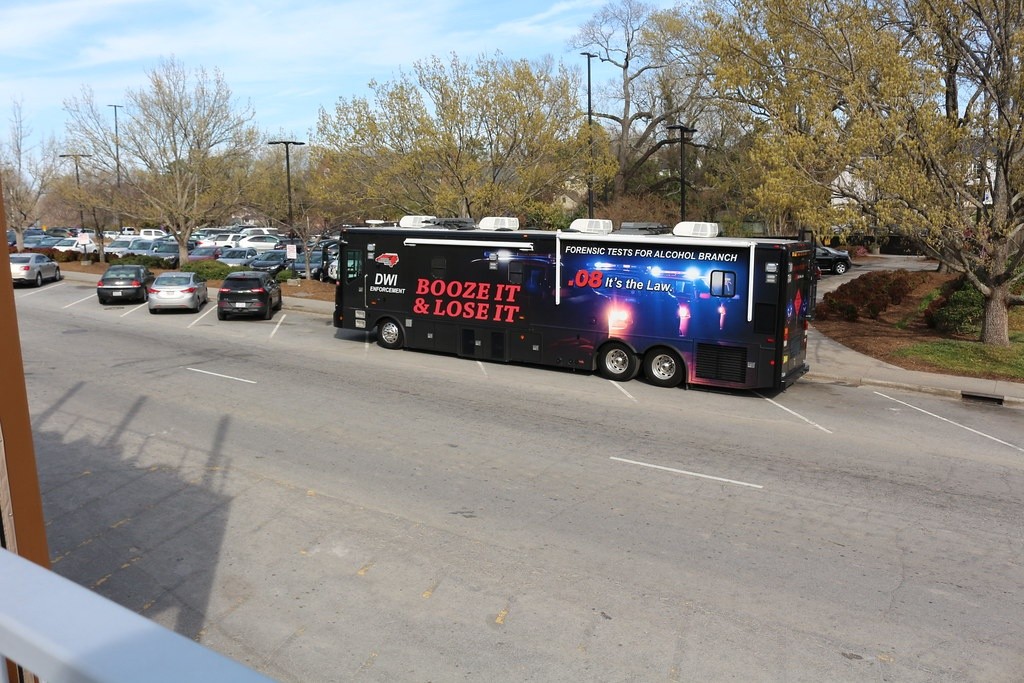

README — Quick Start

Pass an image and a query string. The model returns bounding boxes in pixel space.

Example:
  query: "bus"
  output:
[319,212,817,398]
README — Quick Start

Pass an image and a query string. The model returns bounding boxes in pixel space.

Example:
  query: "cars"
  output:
[213,246,260,271]
[183,245,225,265]
[153,225,338,252]
[6,225,98,255]
[98,261,151,302]
[250,249,289,277]
[104,237,140,255]
[812,245,853,276]
[154,241,187,263]
[100,231,119,240]
[9,251,61,285]
[121,226,138,236]
[149,271,208,315]
[123,239,160,259]
[141,228,166,240]
[282,252,322,283]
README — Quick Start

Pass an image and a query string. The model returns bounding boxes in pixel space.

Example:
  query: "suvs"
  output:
[216,271,282,321]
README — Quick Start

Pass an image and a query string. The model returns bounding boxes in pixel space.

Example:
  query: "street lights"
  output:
[106,103,124,229]
[666,122,697,221]
[54,152,95,225]
[579,50,602,218]
[266,140,307,241]
